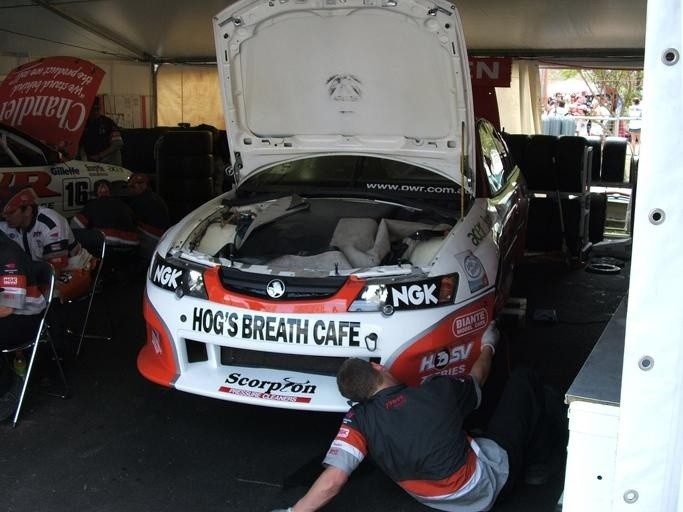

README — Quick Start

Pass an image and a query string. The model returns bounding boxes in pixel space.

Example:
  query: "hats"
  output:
[0,182,38,218]
[93,180,112,198]
[125,173,150,188]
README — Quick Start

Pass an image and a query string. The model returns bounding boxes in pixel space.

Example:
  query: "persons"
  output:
[78,94,125,167]
[538,86,625,149]
[0,186,99,389]
[270,316,539,510]
[627,97,643,156]
[0,228,51,423]
[68,179,143,289]
[120,171,173,258]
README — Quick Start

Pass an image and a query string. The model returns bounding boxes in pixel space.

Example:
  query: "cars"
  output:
[0,56,145,219]
[135,0,530,416]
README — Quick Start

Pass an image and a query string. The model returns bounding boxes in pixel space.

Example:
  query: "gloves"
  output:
[477,318,504,357]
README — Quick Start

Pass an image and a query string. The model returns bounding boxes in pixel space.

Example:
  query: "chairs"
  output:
[53,228,113,357]
[0,259,70,430]
